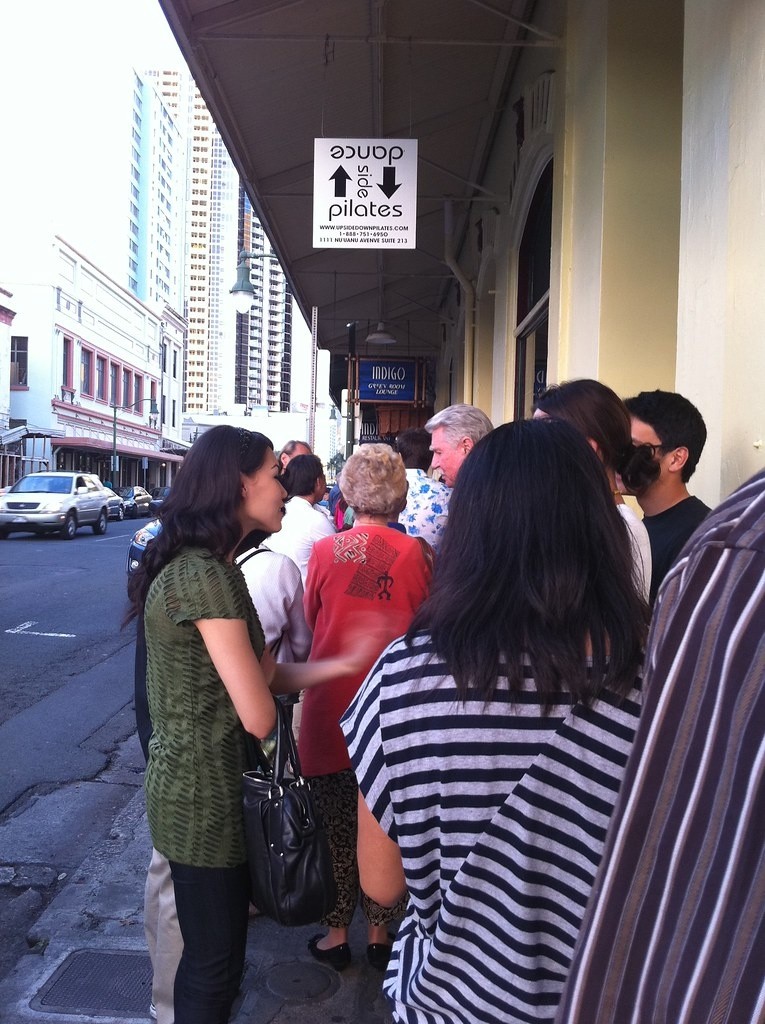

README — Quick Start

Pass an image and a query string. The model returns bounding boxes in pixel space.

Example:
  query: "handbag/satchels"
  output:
[239,696,339,929]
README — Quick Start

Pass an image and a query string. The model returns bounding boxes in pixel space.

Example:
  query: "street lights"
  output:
[109,398,159,494]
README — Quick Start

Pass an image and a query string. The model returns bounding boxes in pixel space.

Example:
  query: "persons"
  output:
[302,442,411,972]
[134,593,185,1024]
[531,379,661,605]
[233,427,455,729]
[339,418,655,1024]
[555,464,765,1024]
[424,403,494,488]
[123,424,373,1024]
[622,390,714,606]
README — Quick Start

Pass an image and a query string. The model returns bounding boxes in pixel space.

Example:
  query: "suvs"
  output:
[0,470,108,540]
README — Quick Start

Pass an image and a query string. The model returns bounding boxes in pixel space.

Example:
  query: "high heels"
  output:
[367,943,390,972]
[306,933,351,973]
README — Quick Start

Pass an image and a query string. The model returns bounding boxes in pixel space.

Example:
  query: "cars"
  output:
[125,500,164,603]
[148,487,171,501]
[104,487,126,521]
[112,486,154,520]
